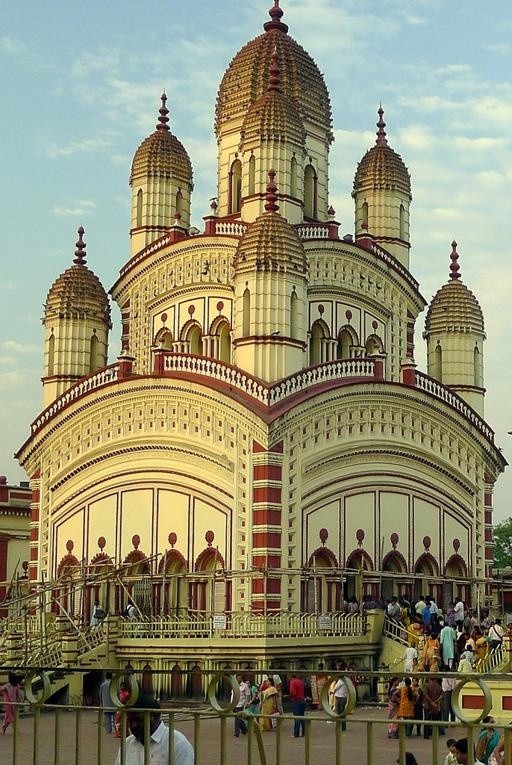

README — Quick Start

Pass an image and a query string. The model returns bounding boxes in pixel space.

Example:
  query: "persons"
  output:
[99,671,196,765]
[233,659,352,738]
[125,601,137,620]
[397,752,417,764]
[0,673,21,736]
[387,654,471,739]
[344,594,512,665]
[445,716,511,765]
[89,599,104,626]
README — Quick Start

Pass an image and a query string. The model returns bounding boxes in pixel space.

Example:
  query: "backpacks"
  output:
[124,605,132,615]
